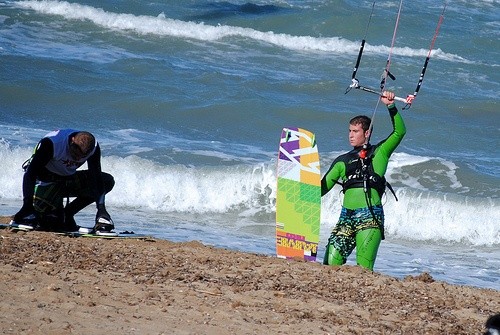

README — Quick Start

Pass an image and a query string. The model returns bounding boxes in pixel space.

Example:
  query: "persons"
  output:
[321,89,408,273]
[12,127,116,231]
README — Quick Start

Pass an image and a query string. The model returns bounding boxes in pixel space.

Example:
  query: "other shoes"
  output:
[63,208,77,232]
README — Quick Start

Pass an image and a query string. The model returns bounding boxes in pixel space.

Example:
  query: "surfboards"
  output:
[0,223,154,241]
[274,126,322,262]
